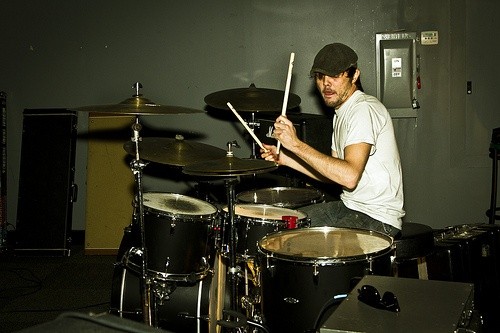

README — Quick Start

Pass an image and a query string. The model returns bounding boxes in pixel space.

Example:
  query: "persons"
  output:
[239,42,405,263]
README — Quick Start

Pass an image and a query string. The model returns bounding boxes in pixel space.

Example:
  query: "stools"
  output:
[391,222,432,279]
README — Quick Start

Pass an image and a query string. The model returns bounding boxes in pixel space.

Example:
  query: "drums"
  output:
[251,225,395,333]
[234,185,327,210]
[216,203,311,262]
[122,190,221,284]
[109,231,226,333]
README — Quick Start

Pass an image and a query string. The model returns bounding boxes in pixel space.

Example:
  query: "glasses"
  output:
[357,284,401,312]
[316,69,349,80]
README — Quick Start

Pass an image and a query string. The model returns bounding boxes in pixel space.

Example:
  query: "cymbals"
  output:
[181,156,279,179]
[123,137,236,168]
[202,86,302,112]
[65,97,209,116]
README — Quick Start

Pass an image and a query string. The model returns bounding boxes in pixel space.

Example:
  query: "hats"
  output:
[311,43,358,76]
